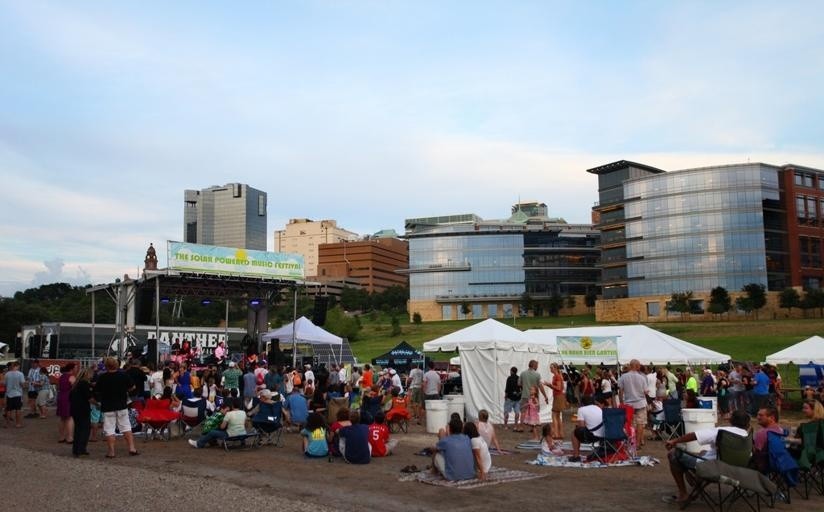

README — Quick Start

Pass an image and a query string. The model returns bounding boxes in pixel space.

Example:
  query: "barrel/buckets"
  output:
[424,394,465,434]
[696,396,718,423]
[681,408,718,460]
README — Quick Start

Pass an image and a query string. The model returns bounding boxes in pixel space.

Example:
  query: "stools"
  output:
[223,433,260,452]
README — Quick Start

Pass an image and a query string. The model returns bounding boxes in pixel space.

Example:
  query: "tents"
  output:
[424,318,558,426]
[763,336,823,366]
[260,316,342,366]
[520,324,732,366]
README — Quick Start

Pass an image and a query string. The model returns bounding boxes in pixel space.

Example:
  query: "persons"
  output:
[497,360,570,445]
[675,360,784,427]
[427,410,509,480]
[56,358,154,458]
[300,360,423,463]
[418,361,461,426]
[559,360,679,467]
[148,338,307,449]
[664,386,823,506]
[0,359,50,427]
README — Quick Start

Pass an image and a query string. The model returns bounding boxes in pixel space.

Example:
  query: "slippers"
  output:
[401,464,421,473]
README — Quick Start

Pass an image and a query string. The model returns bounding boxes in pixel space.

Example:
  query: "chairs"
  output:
[653,399,684,442]
[252,401,283,449]
[385,398,408,434]
[589,408,628,464]
[681,419,824,512]
[144,399,170,442]
